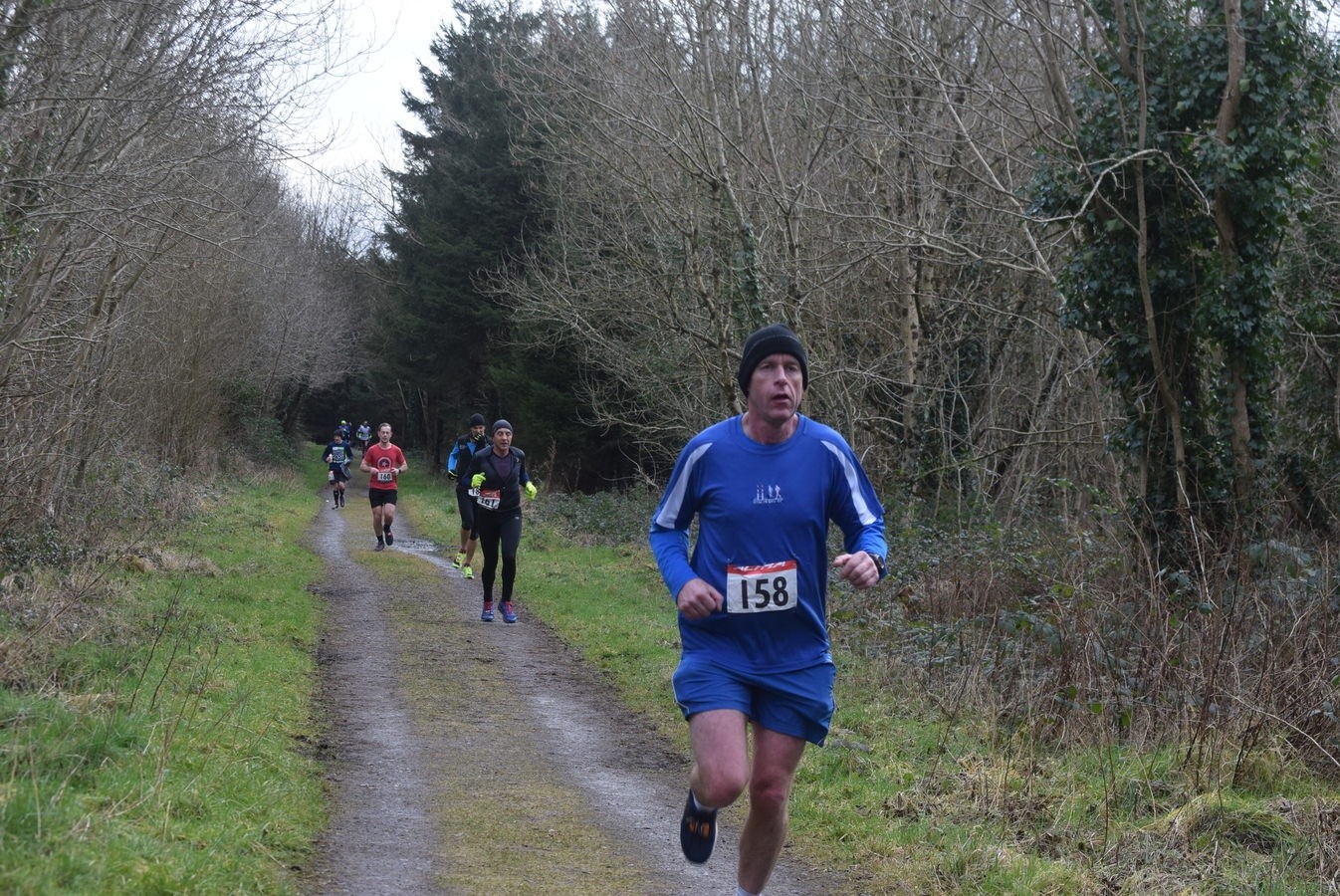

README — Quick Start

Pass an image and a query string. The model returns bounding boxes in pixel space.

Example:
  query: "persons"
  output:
[462,420,537,624]
[447,414,494,580]
[322,420,372,511]
[360,422,409,551]
[646,325,887,896]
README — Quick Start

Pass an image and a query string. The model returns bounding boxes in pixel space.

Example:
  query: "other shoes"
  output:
[340,497,344,507]
[362,455,365,458]
[331,505,338,510]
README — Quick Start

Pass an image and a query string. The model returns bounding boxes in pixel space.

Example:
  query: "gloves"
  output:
[524,481,537,500]
[447,470,458,480]
[471,473,486,488]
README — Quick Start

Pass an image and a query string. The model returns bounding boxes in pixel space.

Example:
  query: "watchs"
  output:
[398,468,402,474]
[867,553,884,575]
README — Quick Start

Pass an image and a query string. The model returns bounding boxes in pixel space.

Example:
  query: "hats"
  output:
[737,325,809,398]
[469,414,486,428]
[341,420,346,424]
[491,420,514,438]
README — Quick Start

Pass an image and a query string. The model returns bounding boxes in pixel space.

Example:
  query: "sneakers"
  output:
[498,601,517,623]
[452,552,467,568]
[463,566,474,580]
[481,601,494,620]
[375,542,385,551]
[383,525,393,546]
[680,789,718,863]
